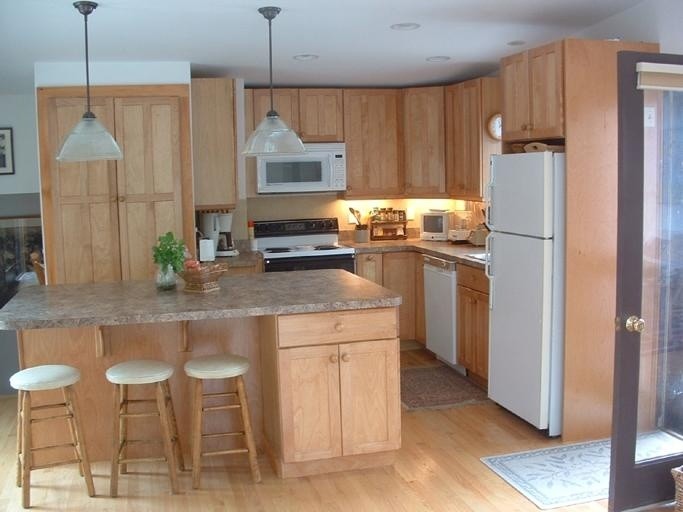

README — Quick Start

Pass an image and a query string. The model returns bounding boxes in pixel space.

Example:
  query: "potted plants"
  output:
[151,232,185,290]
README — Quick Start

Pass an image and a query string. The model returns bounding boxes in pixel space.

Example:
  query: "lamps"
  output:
[241,7,308,156]
[57,0,123,162]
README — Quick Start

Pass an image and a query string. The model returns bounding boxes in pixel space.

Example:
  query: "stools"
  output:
[9,364,95,509]
[106,358,187,495]
[183,354,262,489]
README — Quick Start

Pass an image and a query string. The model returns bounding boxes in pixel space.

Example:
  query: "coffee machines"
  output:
[197,208,240,257]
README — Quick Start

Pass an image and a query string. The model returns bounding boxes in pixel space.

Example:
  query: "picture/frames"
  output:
[0,127,15,175]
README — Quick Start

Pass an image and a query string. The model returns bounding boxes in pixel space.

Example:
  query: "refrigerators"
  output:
[485,152,564,439]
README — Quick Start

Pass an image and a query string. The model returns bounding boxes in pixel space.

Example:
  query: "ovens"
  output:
[263,254,357,275]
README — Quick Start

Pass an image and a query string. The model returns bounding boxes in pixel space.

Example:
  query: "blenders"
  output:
[446,211,473,243]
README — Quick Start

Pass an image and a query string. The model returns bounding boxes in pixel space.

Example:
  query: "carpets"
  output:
[400,364,486,412]
[479,429,683,511]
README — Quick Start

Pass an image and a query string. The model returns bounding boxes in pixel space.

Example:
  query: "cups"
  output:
[199,238,215,262]
[250,239,257,251]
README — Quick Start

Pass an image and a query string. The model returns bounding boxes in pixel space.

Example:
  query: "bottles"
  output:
[248,218,254,240]
[370,208,405,239]
[354,224,370,243]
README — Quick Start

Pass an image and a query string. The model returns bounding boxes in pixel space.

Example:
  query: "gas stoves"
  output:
[259,242,355,259]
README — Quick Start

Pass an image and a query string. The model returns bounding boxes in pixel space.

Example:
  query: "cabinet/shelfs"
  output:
[260,309,401,479]
[500,40,564,143]
[456,263,490,391]
[404,77,502,203]
[190,77,235,209]
[356,252,416,341]
[337,88,405,201]
[36,84,197,285]
[252,88,344,144]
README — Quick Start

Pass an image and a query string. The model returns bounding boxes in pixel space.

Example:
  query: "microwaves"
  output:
[255,141,347,195]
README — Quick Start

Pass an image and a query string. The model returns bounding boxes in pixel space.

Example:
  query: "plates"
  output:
[428,207,449,212]
